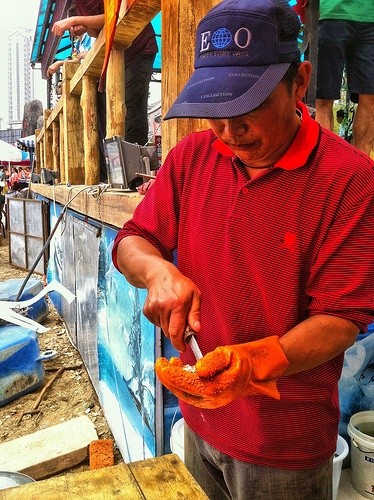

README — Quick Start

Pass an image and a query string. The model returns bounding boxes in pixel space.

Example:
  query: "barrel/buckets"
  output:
[317,434,350,500]
[346,410,374,500]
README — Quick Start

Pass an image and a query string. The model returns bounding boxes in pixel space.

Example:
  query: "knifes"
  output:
[184,323,203,361]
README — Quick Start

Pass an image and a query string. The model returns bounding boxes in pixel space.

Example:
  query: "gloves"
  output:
[154,335,289,410]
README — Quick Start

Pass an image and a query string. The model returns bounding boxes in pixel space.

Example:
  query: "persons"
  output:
[0,166,30,196]
[51,0,159,188]
[46,24,96,99]
[317,0,373,165]
[112,0,374,500]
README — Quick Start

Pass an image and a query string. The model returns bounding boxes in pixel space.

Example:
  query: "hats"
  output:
[163,0,301,121]
